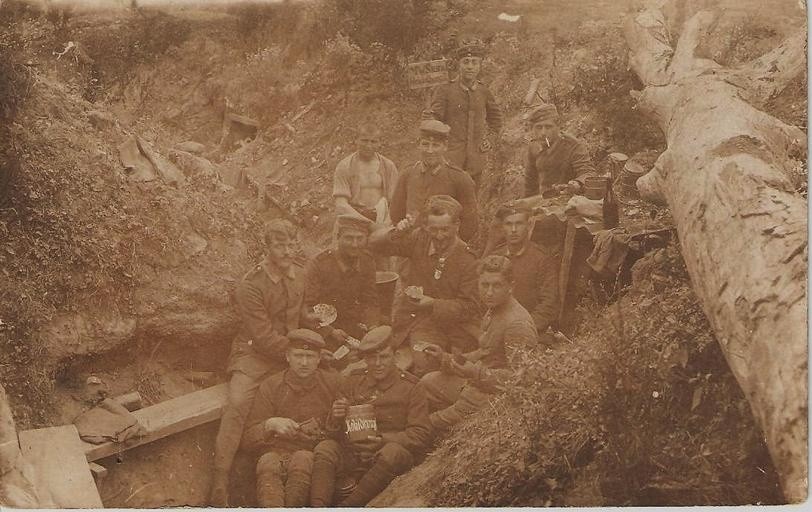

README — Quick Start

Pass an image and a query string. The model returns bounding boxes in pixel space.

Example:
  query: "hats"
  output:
[457,45,487,60]
[494,200,533,219]
[529,104,559,122]
[336,213,372,233]
[428,193,464,214]
[419,120,451,139]
[287,327,327,351]
[358,325,394,355]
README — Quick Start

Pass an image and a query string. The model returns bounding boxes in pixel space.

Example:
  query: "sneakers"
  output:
[211,480,230,508]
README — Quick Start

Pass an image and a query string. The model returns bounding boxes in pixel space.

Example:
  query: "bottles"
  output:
[603,177,620,231]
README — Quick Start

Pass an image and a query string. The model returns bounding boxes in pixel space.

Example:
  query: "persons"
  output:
[420,253,542,441]
[482,103,594,258]
[390,118,479,245]
[420,43,505,201]
[206,216,307,508]
[367,193,481,378]
[295,214,381,373]
[483,197,562,347]
[239,327,344,508]
[331,124,400,243]
[308,324,432,508]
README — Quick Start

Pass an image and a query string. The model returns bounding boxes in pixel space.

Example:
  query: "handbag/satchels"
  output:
[75,398,142,445]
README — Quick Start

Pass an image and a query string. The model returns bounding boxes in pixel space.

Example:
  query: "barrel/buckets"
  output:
[612,161,643,208]
[606,151,628,182]
[583,174,609,199]
[342,405,377,442]
[376,267,399,320]
